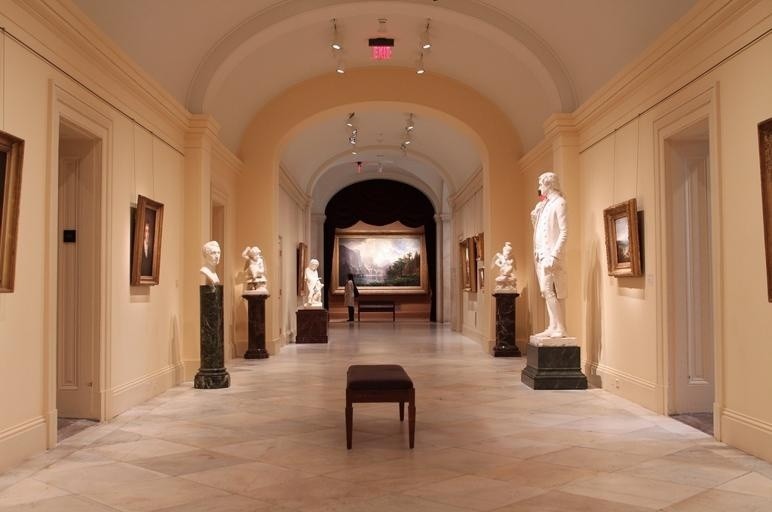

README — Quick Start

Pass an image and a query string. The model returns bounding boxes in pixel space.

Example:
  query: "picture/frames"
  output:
[603,197,641,277]
[1,129,25,294]
[477,266,484,289]
[298,242,308,295]
[474,235,484,260]
[132,194,165,287]
[758,117,772,302]
[459,237,477,292]
[330,229,429,294]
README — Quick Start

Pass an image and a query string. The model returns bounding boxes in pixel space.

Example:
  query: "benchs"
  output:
[358,306,395,322]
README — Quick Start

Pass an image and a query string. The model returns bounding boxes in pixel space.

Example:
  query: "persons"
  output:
[199,240,221,286]
[493,241,517,290]
[241,245,268,288]
[343,272,356,321]
[304,258,324,306]
[530,171,569,339]
[141,220,152,277]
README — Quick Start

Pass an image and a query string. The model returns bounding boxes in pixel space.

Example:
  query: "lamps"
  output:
[331,20,342,52]
[347,129,359,146]
[416,54,425,76]
[370,37,395,59]
[401,136,407,151]
[419,20,432,52]
[336,56,346,75]
[344,112,360,125]
[404,113,415,132]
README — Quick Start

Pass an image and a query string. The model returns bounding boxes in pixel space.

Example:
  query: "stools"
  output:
[345,364,416,449]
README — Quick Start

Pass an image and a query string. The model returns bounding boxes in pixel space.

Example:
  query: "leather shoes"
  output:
[347,320,354,321]
